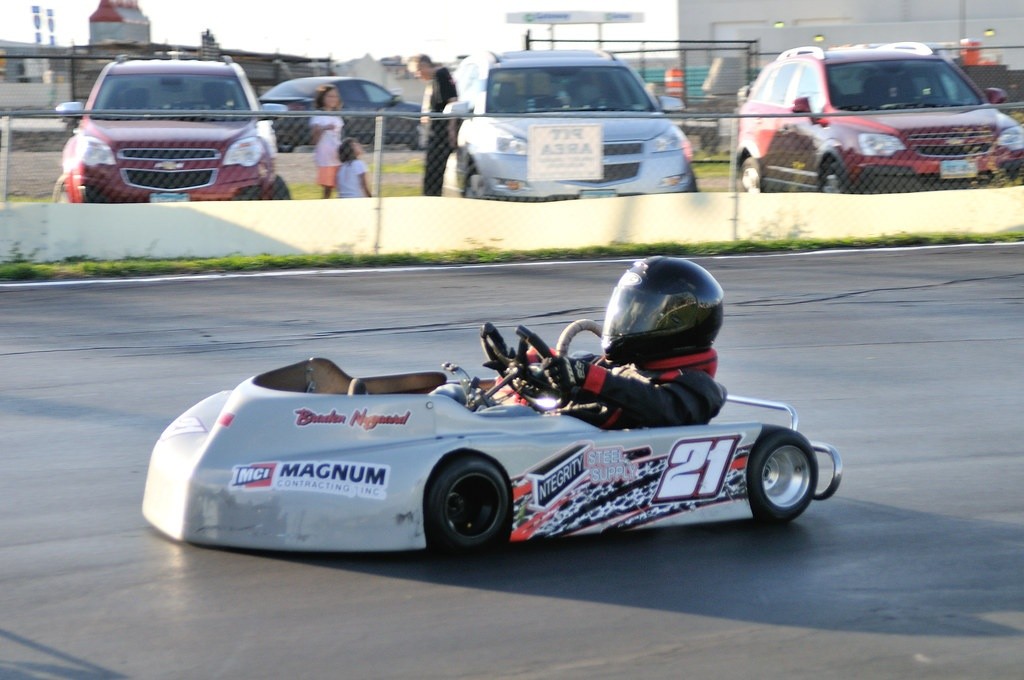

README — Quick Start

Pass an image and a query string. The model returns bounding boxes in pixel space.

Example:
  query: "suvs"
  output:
[736,39,1024,195]
[51,52,292,203]
[443,45,700,202]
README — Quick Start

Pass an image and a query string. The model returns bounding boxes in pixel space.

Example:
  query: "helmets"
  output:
[601,255,723,367]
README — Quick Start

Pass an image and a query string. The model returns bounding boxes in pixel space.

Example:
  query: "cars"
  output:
[254,75,432,153]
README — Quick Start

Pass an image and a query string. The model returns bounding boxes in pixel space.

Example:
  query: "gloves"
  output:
[512,362,550,395]
[541,357,588,391]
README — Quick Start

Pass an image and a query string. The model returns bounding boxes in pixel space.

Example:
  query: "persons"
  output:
[407,54,460,196]
[308,84,344,198]
[428,255,728,429]
[335,138,372,198]
